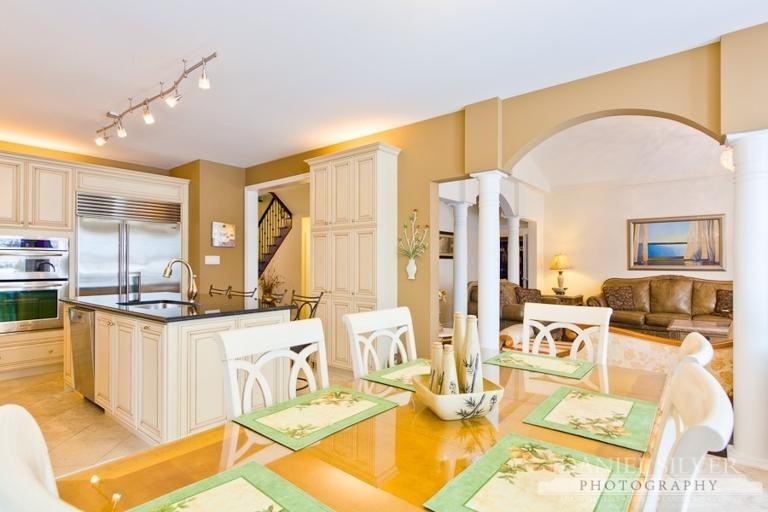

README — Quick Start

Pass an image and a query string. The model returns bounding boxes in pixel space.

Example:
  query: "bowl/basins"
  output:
[411,372,505,422]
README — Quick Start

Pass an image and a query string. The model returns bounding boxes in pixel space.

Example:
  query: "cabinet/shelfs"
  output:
[0,157,72,231]
[303,141,403,372]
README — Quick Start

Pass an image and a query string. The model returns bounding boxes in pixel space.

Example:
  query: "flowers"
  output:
[259,260,288,308]
[398,207,429,258]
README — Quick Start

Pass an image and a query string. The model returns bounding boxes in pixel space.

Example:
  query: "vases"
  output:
[406,259,417,279]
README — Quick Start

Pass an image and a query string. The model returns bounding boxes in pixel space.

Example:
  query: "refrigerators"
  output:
[70,189,184,298]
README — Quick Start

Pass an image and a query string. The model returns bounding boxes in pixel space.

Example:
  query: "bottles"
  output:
[427,311,484,399]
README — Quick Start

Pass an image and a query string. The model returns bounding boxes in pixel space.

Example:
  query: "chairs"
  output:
[467,279,560,326]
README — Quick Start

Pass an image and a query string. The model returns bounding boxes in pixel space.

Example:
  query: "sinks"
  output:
[115,300,192,310]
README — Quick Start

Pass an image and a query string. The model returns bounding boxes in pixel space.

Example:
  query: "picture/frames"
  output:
[438,231,454,259]
[627,214,727,271]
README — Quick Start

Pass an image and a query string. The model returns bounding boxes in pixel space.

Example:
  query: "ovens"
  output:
[0,232,69,335]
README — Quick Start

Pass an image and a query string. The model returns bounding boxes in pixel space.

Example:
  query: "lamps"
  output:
[95,52,216,146]
[548,252,573,295]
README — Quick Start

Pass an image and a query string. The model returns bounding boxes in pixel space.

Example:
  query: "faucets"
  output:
[162,257,198,303]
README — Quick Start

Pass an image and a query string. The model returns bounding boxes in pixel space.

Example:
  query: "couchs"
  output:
[586,275,734,330]
